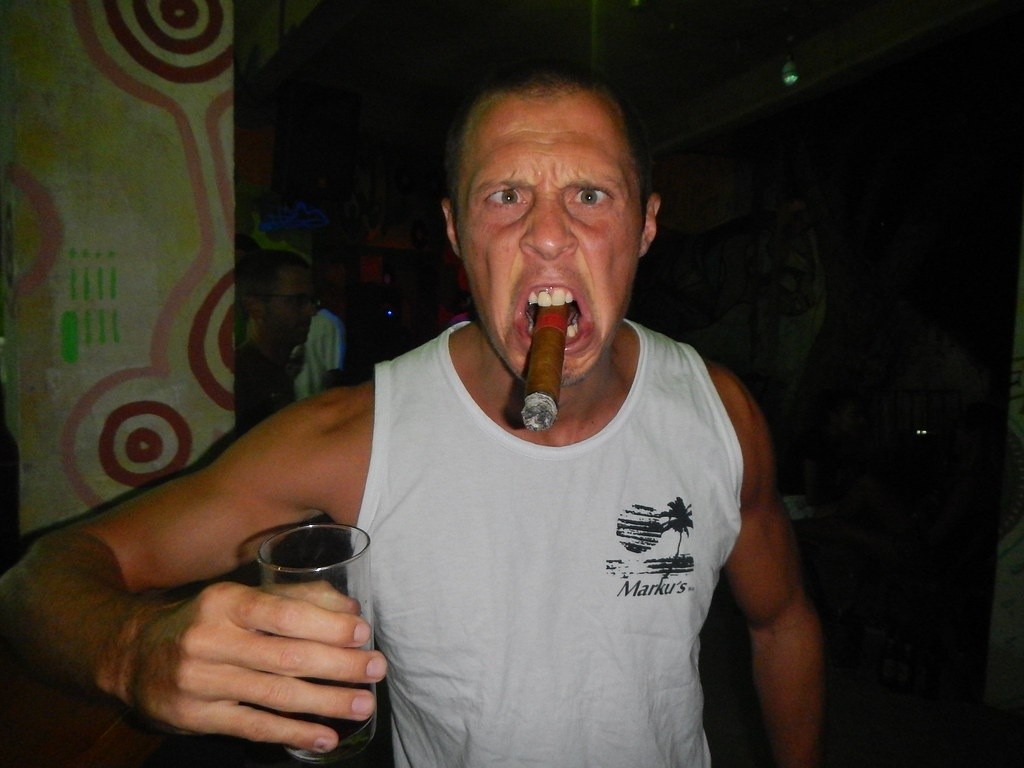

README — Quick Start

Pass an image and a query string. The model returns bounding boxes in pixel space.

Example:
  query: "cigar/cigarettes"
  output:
[519,304,572,430]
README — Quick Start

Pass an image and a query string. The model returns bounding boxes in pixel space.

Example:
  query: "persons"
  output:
[0,65,823,767]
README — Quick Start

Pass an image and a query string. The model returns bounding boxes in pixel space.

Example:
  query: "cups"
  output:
[256,524,376,764]
